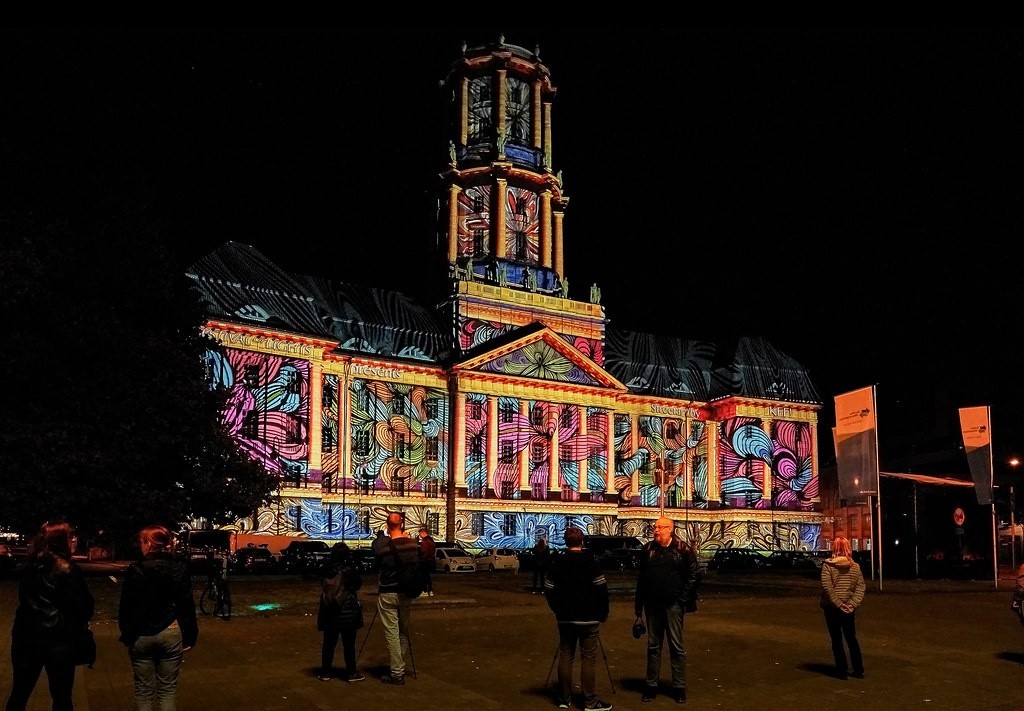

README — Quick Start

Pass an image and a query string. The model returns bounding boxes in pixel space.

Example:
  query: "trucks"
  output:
[182,529,232,575]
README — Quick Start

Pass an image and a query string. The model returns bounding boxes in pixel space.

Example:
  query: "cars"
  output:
[230,547,277,575]
[340,549,381,577]
[518,547,643,574]
[433,547,477,575]
[708,547,872,578]
[474,548,520,576]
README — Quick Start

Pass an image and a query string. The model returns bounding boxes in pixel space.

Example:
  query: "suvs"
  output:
[277,540,336,578]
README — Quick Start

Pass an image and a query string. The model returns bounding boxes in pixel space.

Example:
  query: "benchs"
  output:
[698,563,709,574]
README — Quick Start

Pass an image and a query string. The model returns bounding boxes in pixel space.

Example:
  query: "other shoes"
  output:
[380,674,405,685]
[584,700,613,711]
[557,697,572,709]
[419,590,429,597]
[851,672,865,679]
[317,671,331,681]
[429,590,435,597]
[836,672,848,680]
[348,673,367,682]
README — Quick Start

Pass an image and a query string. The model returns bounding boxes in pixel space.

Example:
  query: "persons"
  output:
[317,541,366,682]
[371,513,424,684]
[532,539,549,594]
[544,528,613,711]
[821,537,866,680]
[5,521,94,711]
[417,529,435,597]
[1012,563,1024,614]
[372,530,390,584]
[118,525,199,711]
[634,518,698,703]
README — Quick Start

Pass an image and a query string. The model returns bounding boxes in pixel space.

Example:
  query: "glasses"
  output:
[652,525,670,530]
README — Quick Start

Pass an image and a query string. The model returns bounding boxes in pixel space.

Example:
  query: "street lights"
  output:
[1008,456,1023,570]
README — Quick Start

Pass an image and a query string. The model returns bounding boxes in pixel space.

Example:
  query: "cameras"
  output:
[632,624,646,639]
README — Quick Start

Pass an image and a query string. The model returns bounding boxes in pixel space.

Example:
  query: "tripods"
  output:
[357,610,418,681]
[545,636,616,695]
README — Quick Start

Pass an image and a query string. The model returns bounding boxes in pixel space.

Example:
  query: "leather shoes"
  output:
[673,688,688,703]
[641,686,657,701]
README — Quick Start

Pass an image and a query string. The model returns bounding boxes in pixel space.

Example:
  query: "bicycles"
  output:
[200,558,232,622]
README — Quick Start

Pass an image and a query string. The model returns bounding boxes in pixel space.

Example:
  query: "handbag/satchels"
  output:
[30,626,97,670]
[399,568,424,599]
